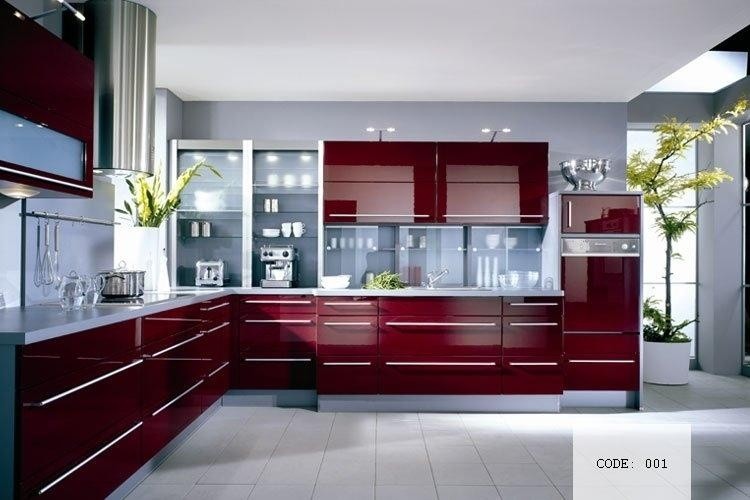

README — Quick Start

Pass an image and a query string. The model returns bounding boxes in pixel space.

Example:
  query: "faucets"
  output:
[426,268,450,287]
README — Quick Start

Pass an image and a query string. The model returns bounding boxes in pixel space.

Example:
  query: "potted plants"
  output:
[114,158,223,291]
[622,93,749,389]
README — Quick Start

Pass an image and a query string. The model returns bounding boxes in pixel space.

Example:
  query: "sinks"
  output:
[427,287,493,292]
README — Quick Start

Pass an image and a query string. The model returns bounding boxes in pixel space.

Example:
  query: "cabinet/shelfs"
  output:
[376,296,502,417]
[0,6,96,199]
[436,141,548,226]
[501,296,565,415]
[326,226,398,288]
[0,316,145,499]
[548,191,644,237]
[315,294,376,413]
[170,139,246,289]
[467,225,541,288]
[137,304,202,463]
[247,139,319,294]
[242,290,317,408]
[318,141,438,223]
[201,294,246,424]
[397,224,467,288]
[547,256,642,413]
